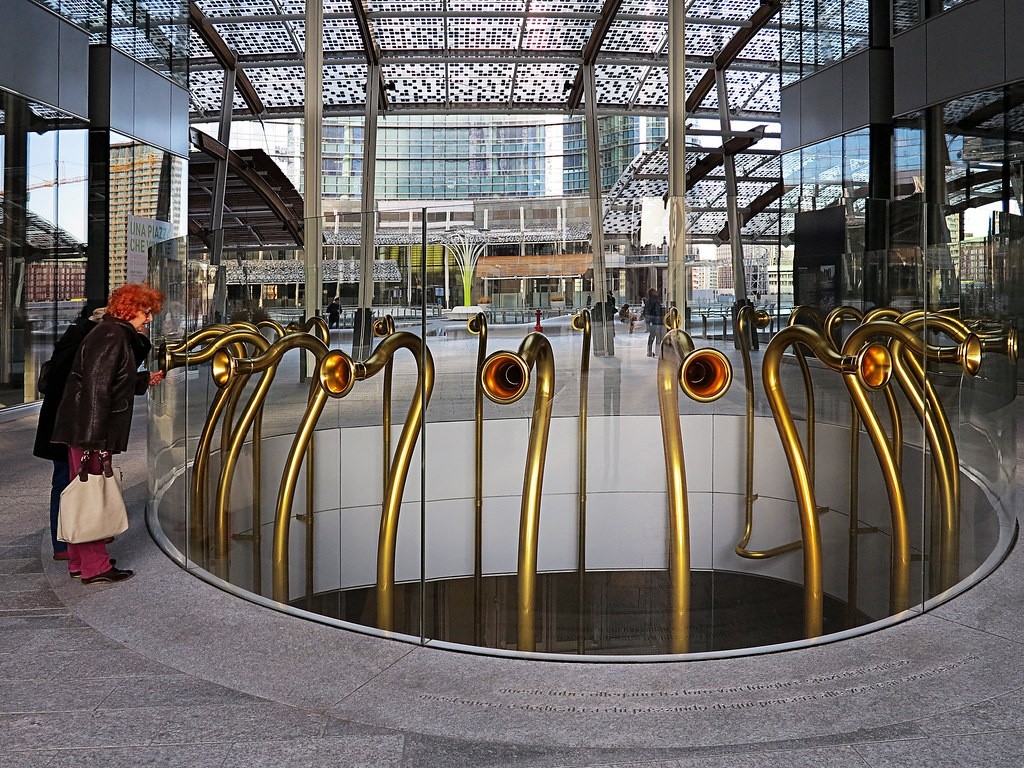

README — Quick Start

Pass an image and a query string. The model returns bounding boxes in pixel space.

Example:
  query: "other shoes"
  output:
[647,353,655,357]
[104,537,114,543]
[53,550,69,560]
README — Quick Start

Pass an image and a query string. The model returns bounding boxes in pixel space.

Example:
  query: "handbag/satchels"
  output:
[37,360,50,394]
[57,450,128,544]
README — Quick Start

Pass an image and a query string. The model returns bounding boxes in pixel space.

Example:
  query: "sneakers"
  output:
[81,566,134,585]
[69,558,116,578]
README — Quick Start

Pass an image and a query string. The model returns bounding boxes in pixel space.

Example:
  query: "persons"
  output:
[757,291,762,303]
[30,300,153,565]
[48,283,165,585]
[587,288,665,357]
[327,297,342,329]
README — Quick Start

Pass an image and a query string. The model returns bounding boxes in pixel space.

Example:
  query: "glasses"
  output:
[140,309,152,317]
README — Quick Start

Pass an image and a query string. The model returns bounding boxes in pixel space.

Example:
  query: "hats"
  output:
[334,297,342,301]
[607,290,613,295]
